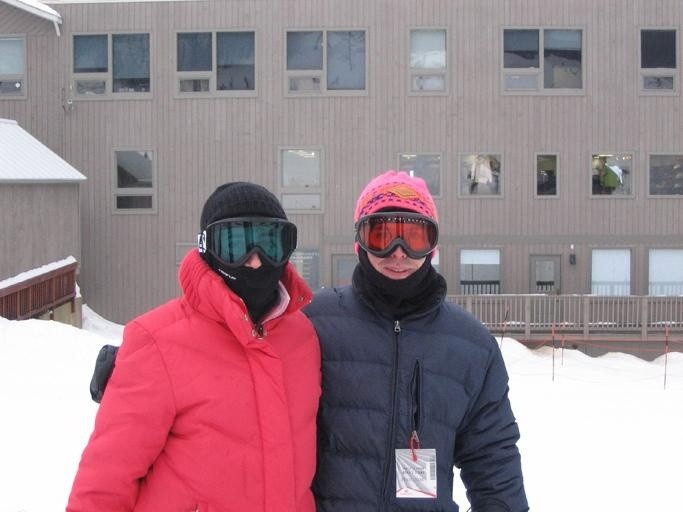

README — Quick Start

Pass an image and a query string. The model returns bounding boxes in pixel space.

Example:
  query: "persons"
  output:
[64,179,324,512]
[88,168,531,511]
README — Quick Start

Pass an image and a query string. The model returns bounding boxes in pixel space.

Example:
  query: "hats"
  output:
[201,182,290,236]
[352,171,439,258]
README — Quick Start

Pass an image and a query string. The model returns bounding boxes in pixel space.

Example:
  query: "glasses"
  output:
[353,211,438,258]
[199,217,299,268]
